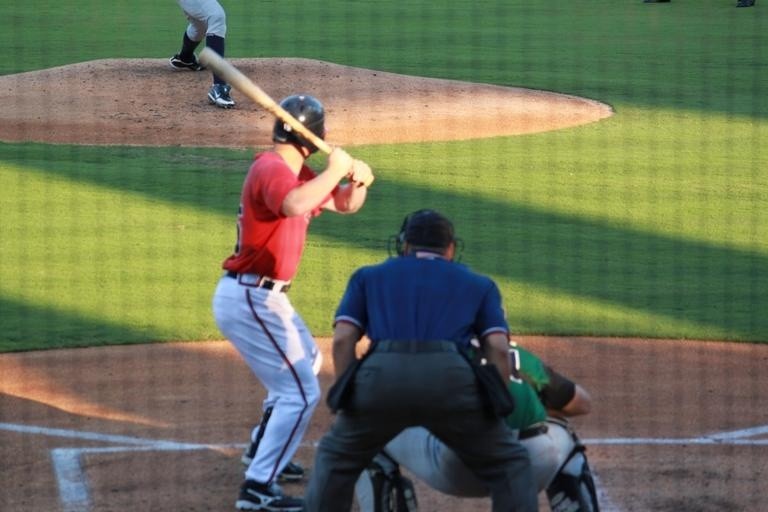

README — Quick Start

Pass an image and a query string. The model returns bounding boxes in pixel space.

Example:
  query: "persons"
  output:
[301,207,539,511]
[210,93,375,511]
[168,1,236,109]
[356,340,603,512]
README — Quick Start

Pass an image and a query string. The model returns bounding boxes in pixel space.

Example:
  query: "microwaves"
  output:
[199,46,376,190]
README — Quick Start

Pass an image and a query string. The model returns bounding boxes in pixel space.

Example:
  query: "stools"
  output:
[518,422,547,440]
[226,270,291,293]
[373,338,458,352]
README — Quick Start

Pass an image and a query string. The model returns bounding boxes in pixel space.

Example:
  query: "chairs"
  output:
[241,441,304,480]
[236,479,304,512]
[170,51,208,71]
[206,83,236,108]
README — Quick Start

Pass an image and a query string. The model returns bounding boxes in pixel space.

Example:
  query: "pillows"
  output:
[388,208,464,262]
[272,94,324,154]
